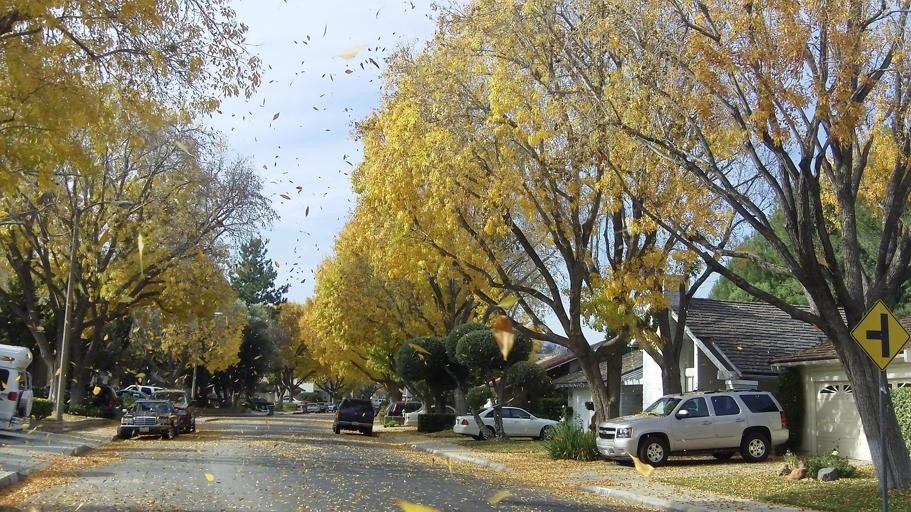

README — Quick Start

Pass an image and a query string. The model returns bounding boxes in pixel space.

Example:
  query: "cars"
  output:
[87,383,198,440]
[206,392,275,415]
[386,401,458,426]
[453,405,563,441]
[305,401,335,413]
[275,397,303,407]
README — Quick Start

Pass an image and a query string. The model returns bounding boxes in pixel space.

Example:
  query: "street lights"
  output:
[56,199,134,423]
[190,311,224,401]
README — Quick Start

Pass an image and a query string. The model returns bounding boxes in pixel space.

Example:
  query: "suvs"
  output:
[332,398,375,436]
[595,388,791,467]
[0,342,34,431]
